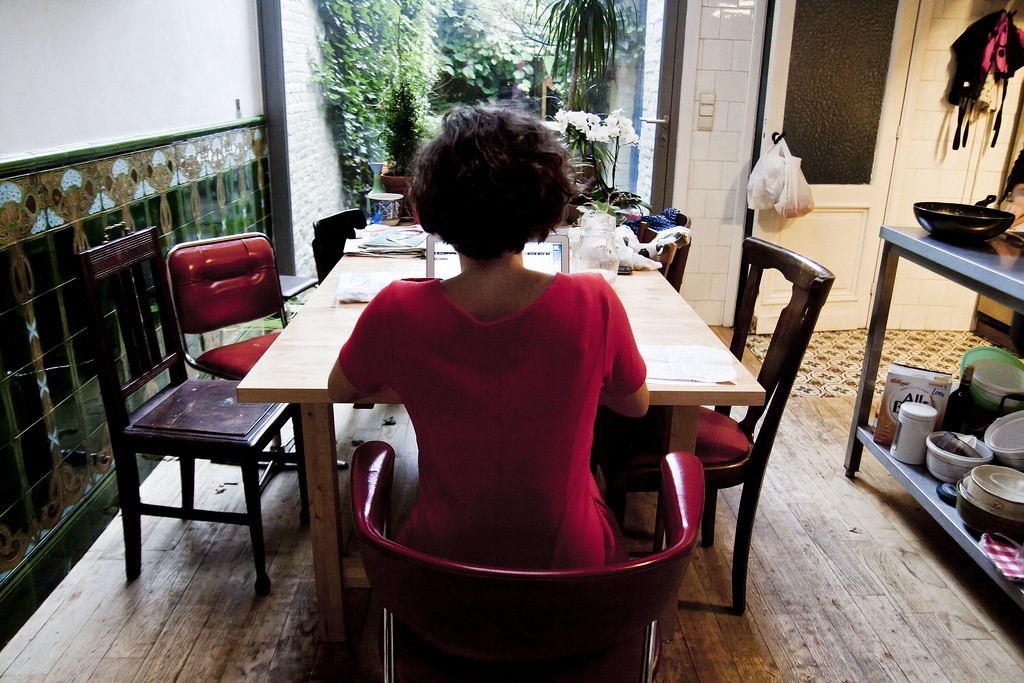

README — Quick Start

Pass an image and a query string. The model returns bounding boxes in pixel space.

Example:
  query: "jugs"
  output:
[572,210,619,287]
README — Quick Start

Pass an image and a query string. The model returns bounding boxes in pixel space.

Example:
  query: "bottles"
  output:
[942,364,975,431]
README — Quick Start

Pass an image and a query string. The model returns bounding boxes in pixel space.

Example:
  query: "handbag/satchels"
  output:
[747,135,815,219]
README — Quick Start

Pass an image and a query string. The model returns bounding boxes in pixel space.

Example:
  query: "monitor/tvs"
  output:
[426,231,569,279]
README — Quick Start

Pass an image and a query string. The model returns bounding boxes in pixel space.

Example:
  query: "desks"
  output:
[236,262,768,648]
[841,226,1024,611]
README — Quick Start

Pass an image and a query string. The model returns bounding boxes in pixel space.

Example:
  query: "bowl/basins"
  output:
[985,409,1024,470]
[957,464,1024,536]
[961,346,1024,412]
[926,431,993,483]
[369,193,404,225]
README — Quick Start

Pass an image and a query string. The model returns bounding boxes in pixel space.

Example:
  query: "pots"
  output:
[913,195,1015,242]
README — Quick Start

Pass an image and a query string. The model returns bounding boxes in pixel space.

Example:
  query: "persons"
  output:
[328,106,648,572]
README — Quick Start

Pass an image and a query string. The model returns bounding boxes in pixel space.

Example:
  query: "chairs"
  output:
[347,440,706,682]
[312,207,368,289]
[72,225,311,597]
[633,219,690,293]
[163,231,349,498]
[593,236,835,618]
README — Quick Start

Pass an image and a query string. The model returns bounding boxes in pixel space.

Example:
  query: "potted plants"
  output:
[380,82,425,221]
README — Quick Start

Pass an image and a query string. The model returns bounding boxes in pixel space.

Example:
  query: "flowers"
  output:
[551,105,641,200]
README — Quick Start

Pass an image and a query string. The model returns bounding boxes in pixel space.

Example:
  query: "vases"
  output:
[369,193,404,226]
[366,162,384,214]
